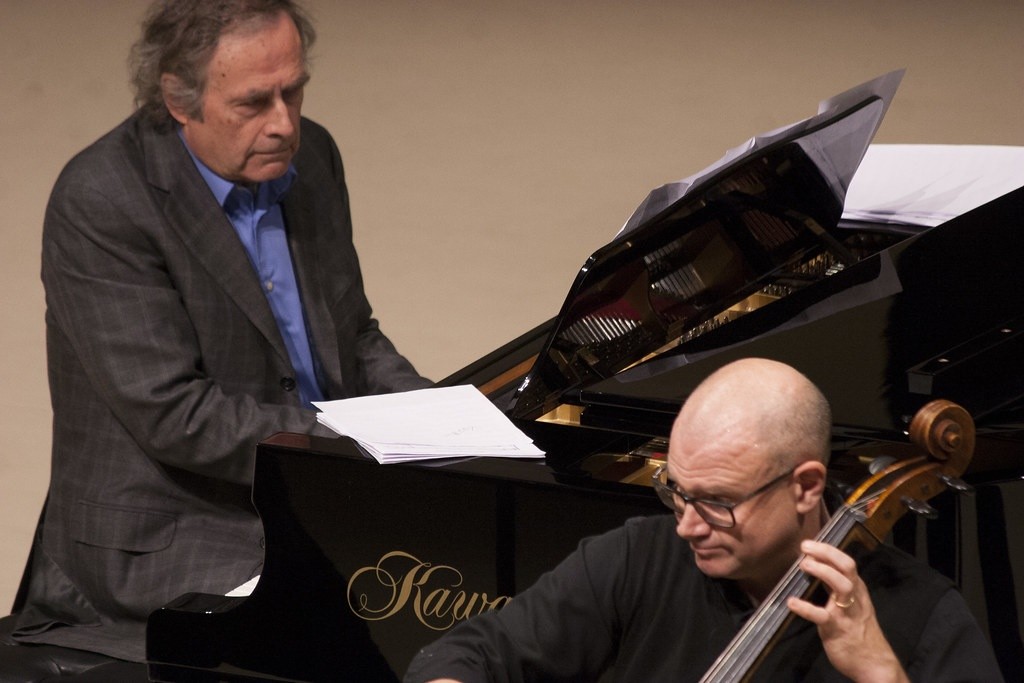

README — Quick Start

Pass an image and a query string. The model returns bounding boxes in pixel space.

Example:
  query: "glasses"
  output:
[651,459,794,529]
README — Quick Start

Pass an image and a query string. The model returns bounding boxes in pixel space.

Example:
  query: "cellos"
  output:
[695,399,978,683]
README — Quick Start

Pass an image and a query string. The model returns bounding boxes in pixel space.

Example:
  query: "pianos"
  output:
[145,95,1024,683]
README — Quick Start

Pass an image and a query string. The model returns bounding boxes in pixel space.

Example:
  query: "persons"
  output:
[9,1,436,663]
[405,359,1001,683]
